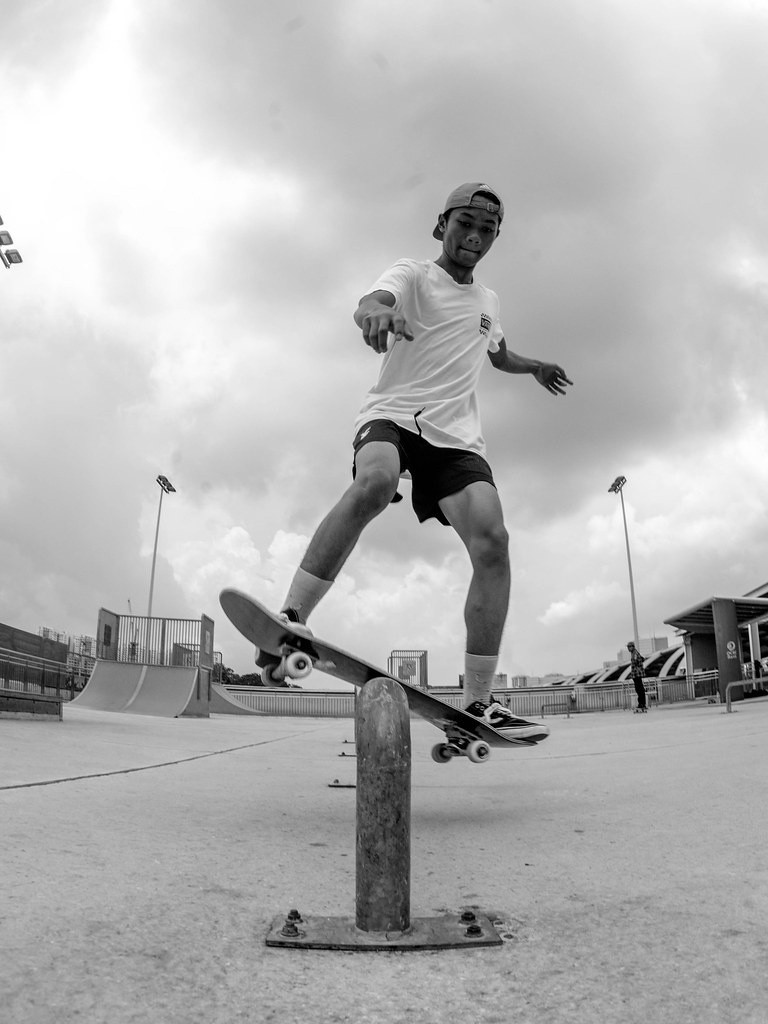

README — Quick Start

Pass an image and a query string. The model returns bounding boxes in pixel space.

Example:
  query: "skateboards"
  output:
[221,590,540,763]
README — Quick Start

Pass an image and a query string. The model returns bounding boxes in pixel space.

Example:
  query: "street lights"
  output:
[607,475,641,654]
[144,475,177,666]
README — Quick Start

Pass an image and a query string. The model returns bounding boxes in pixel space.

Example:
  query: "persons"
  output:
[627,641,647,708]
[253,183,572,744]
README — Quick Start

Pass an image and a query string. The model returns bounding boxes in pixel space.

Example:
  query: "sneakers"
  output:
[255,608,314,669]
[464,695,549,742]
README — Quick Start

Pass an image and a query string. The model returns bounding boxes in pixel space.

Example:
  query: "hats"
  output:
[433,182,504,241]
